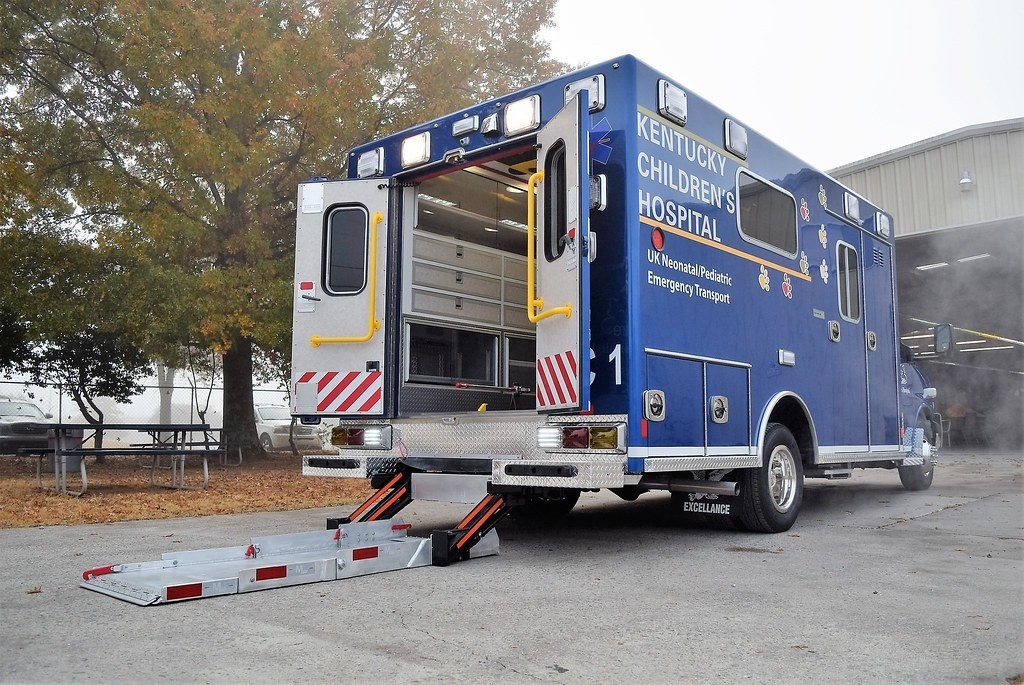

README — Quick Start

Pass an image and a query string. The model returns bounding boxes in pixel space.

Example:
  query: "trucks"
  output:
[77,52,952,609]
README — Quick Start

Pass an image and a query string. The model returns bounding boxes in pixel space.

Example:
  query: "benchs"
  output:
[17,449,227,497]
[129,441,249,470]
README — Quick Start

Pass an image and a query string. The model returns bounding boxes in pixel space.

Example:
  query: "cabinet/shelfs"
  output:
[401,228,536,396]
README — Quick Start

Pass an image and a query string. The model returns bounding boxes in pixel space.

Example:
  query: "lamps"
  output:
[959,172,975,192]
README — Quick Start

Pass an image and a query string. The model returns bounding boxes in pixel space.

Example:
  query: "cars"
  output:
[255,405,324,452]
[0,396,56,452]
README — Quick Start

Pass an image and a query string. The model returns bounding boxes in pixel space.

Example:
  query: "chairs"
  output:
[956,414,998,447]
[933,413,951,447]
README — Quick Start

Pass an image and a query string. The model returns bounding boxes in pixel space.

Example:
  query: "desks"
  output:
[29,423,211,494]
[942,417,985,446]
[138,427,226,468]
